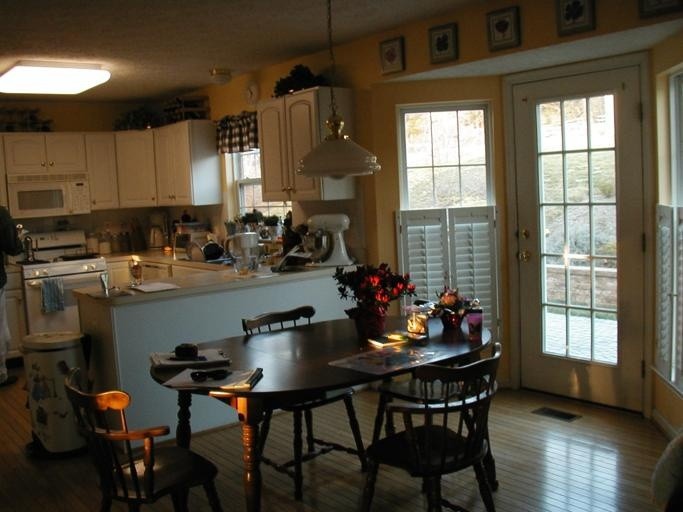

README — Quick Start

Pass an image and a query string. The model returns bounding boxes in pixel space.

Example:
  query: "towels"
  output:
[42,277,65,314]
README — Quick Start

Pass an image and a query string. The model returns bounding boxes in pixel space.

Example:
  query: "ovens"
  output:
[23,271,110,335]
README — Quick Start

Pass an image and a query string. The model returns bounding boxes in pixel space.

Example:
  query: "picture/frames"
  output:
[426,22,458,65]
[486,6,520,52]
[377,36,407,75]
[555,0,596,37]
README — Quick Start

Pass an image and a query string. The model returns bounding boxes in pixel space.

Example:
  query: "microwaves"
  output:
[7,181,92,220]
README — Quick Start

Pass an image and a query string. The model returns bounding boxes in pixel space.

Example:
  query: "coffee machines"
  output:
[305,213,355,267]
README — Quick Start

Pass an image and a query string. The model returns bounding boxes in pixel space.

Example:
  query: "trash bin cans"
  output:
[18,331,88,453]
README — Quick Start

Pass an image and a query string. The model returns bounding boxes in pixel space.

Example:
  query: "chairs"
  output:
[359,343,502,510]
[383,299,443,435]
[63,367,223,512]
[241,306,366,499]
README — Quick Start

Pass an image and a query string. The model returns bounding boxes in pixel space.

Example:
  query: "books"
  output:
[367,327,412,349]
[150,348,264,393]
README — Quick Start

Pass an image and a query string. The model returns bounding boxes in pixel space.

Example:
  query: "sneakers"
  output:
[0,375,18,389]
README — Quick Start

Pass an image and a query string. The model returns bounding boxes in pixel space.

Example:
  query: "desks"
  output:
[151,316,498,510]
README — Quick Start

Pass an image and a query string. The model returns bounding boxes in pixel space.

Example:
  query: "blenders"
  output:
[224,232,258,273]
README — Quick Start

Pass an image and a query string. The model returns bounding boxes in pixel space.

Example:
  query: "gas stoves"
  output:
[16,250,98,265]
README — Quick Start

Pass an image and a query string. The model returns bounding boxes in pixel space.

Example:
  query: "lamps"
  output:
[293,0,381,179]
[0,61,112,97]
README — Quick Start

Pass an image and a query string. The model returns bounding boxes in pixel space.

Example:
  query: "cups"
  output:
[127,260,143,286]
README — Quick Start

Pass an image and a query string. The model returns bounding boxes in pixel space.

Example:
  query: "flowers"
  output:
[332,262,419,316]
[425,286,478,318]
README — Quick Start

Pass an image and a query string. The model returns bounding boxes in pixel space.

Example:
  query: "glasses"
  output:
[191,369,232,381]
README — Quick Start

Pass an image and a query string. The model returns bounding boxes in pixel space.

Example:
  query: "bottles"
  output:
[466,307,483,343]
[85,231,132,254]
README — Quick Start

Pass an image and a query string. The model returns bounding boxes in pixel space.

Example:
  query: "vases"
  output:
[348,307,387,337]
[440,314,465,329]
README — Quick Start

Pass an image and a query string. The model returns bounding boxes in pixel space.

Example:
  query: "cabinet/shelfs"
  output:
[140,262,171,278]
[152,121,192,208]
[258,88,358,201]
[173,267,215,276]
[83,134,119,211]
[1,134,88,174]
[114,131,157,212]
[2,267,29,359]
